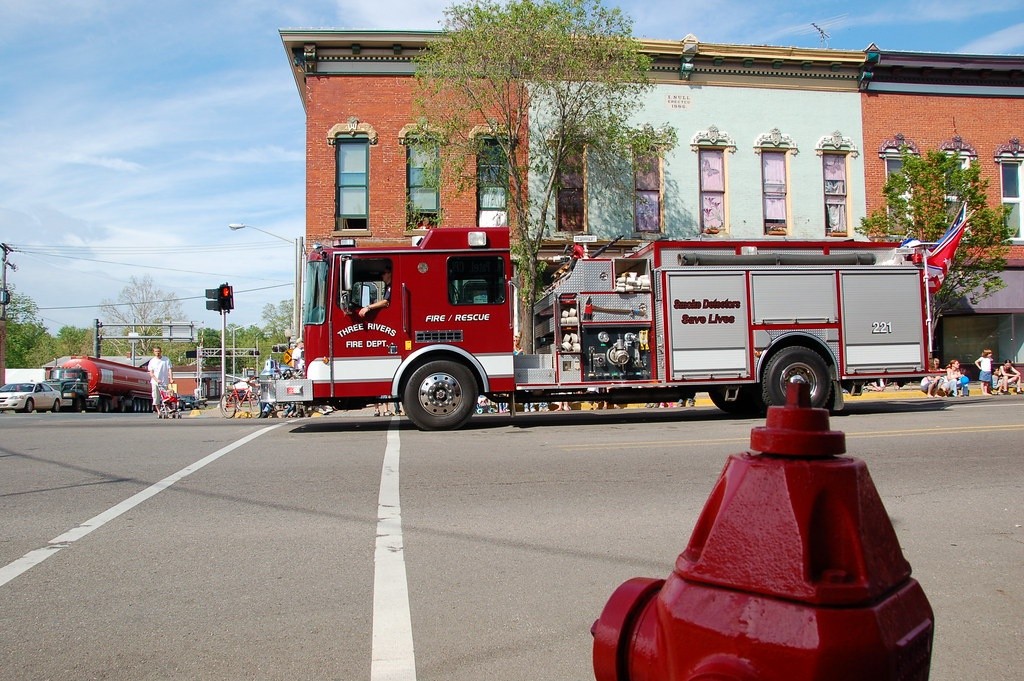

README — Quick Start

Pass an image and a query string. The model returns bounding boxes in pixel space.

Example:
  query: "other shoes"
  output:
[261,411,269,417]
[383,410,392,416]
[926,392,961,398]
[603,406,607,410]
[645,404,674,408]
[983,390,1024,396]
[158,412,162,418]
[82,410,86,414]
[689,402,695,407]
[539,408,549,412]
[281,411,315,417]
[614,406,621,410]
[495,408,510,413]
[395,409,402,415]
[373,409,380,417]
[677,403,686,407]
[525,408,536,412]
[555,407,571,411]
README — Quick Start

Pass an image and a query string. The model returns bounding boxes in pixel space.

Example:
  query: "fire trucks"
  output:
[272,222,944,433]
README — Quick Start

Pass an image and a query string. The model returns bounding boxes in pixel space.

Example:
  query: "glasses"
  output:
[381,270,391,274]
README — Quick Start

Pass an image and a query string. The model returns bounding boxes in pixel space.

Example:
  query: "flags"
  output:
[900,204,967,294]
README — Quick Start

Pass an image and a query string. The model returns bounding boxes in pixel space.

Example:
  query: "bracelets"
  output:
[366,305,372,310]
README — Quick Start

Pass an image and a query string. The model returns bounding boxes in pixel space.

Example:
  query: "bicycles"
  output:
[219,376,262,420]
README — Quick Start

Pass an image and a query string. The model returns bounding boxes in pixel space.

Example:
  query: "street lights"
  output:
[229,223,304,341]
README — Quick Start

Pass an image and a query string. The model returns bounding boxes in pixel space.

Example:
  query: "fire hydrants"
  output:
[588,380,936,681]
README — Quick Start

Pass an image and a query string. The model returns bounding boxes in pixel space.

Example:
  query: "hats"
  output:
[296,338,303,344]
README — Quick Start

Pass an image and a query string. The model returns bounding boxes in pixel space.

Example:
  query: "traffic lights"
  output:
[219,285,234,312]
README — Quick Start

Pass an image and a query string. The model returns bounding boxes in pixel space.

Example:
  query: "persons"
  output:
[645,397,695,408]
[358,265,392,317]
[920,358,969,398]
[291,337,305,419]
[975,348,1022,395]
[416,216,435,229]
[499,403,550,412]
[373,402,394,417]
[148,345,176,419]
[553,402,571,411]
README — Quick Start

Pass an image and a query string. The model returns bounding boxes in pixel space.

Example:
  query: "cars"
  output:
[181,395,200,410]
[0,382,62,414]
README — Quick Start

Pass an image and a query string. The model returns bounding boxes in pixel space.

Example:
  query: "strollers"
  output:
[156,380,180,420]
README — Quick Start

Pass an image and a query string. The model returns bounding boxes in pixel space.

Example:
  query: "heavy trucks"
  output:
[44,354,156,414]
